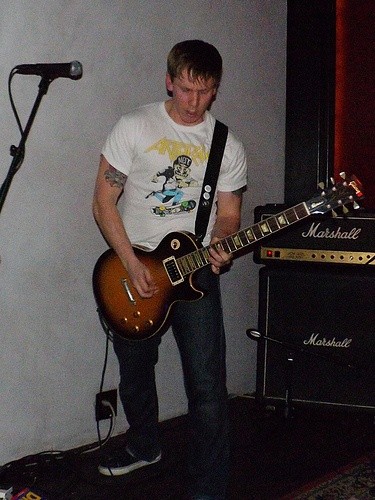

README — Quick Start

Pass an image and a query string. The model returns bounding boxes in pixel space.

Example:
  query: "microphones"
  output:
[16,60,83,80]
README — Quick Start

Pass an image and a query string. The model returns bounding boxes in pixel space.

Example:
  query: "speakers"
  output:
[255,260,375,420]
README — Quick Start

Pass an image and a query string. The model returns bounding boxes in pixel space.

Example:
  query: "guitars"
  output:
[90,169,370,344]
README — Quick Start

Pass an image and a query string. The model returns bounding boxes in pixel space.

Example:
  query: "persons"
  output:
[91,38,249,497]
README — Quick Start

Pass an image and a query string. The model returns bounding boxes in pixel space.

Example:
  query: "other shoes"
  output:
[100,441,164,474]
